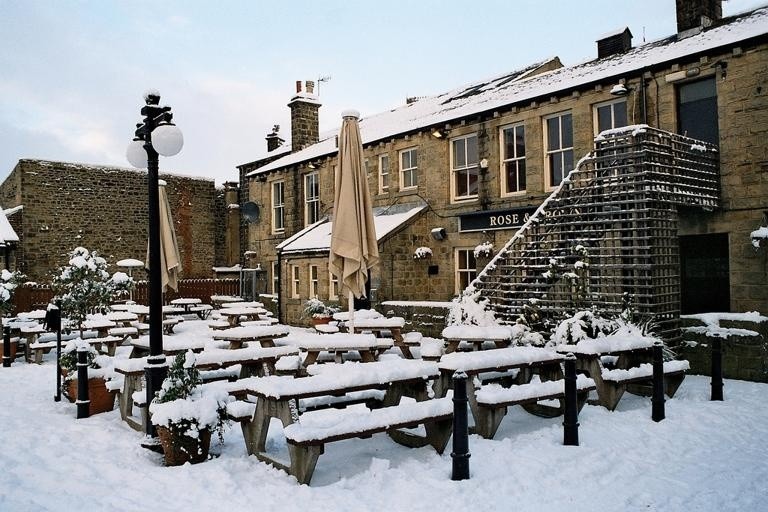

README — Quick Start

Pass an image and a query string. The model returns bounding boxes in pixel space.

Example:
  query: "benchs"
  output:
[0,298,692,485]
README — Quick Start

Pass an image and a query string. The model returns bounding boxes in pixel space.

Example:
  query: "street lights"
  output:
[125,87,188,457]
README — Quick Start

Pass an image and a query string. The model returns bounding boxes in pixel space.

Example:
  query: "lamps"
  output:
[432,128,451,140]
[307,162,323,169]
[610,85,633,97]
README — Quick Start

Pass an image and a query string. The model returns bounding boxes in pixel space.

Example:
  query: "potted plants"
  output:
[0,269,28,364]
[46,245,137,418]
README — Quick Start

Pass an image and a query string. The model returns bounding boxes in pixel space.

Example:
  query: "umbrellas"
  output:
[327,108,380,335]
[144,179,183,294]
